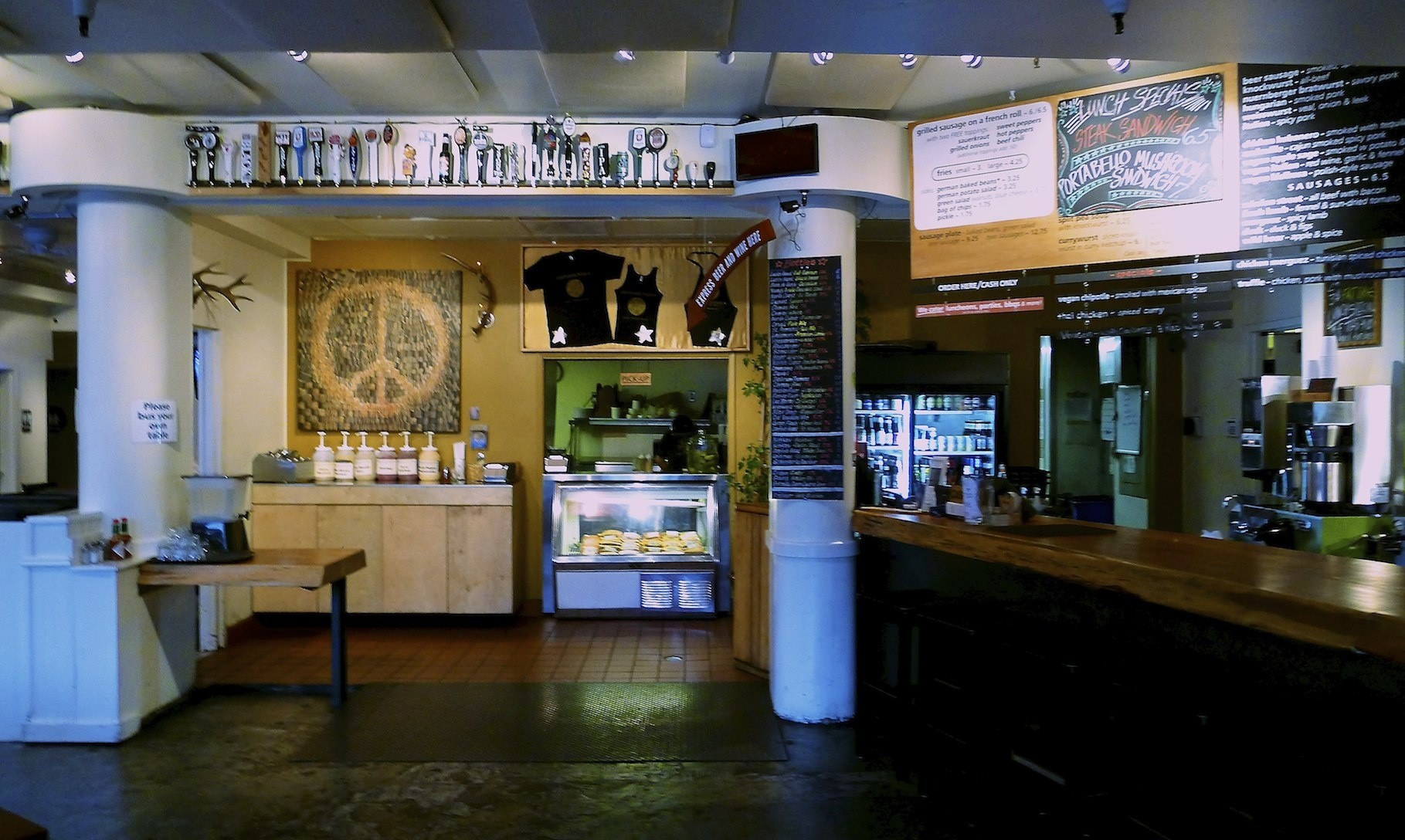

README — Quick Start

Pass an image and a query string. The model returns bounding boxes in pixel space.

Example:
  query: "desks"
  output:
[140,548,367,683]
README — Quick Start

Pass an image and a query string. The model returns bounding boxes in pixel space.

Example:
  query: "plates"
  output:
[594,461,634,473]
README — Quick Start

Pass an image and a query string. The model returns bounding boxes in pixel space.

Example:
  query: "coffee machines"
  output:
[1276,385,1393,518]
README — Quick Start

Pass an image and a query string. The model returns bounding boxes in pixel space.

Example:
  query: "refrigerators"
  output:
[856,342,1010,510]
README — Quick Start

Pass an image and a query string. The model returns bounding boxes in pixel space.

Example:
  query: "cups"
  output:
[611,406,620,418]
[156,524,206,561]
[626,400,640,418]
[1304,336,1339,401]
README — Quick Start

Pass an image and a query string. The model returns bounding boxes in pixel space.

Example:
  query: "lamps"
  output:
[64,50,84,65]
[809,52,833,66]
[613,50,635,66]
[899,53,918,70]
[718,50,735,65]
[961,55,983,69]
[1107,58,1130,74]
[288,50,308,63]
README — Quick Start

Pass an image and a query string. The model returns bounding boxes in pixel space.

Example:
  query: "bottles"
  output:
[80,517,132,564]
[685,429,719,473]
[856,415,991,488]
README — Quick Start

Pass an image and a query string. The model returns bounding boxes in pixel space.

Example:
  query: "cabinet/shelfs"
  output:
[856,393,998,502]
[251,482,522,623]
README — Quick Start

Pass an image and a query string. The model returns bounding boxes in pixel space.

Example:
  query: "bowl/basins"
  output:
[572,408,593,418]
